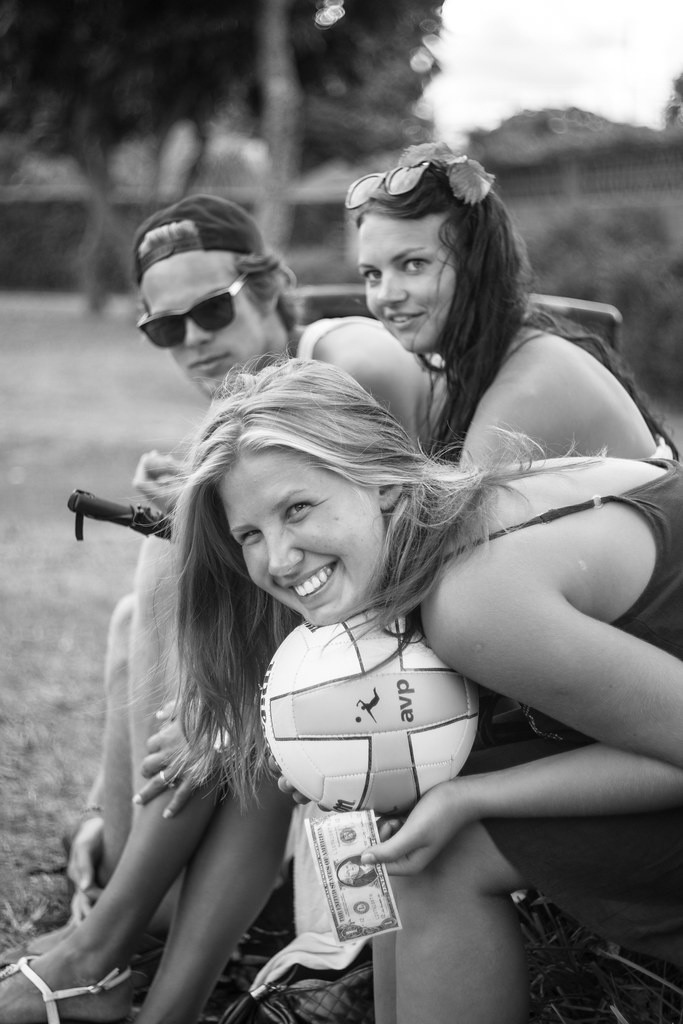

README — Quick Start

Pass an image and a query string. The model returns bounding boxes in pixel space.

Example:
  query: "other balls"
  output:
[257,603,478,816]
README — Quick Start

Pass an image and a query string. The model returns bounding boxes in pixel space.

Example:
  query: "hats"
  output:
[132,194,264,285]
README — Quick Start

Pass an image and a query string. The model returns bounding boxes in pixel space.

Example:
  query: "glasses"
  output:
[137,272,250,347]
[345,161,449,210]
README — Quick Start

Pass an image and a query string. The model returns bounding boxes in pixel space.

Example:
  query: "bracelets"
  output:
[79,805,102,816]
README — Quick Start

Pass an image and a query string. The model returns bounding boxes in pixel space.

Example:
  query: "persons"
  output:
[0,144,683,1024]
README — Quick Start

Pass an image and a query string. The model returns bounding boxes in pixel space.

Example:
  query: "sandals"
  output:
[0,956,131,1024]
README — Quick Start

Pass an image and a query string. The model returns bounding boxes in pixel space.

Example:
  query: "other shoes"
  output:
[227,926,293,963]
[133,933,166,965]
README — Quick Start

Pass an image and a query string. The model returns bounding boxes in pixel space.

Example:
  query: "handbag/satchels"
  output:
[219,961,376,1024]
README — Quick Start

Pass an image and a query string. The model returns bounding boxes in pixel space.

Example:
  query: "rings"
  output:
[159,770,175,790]
